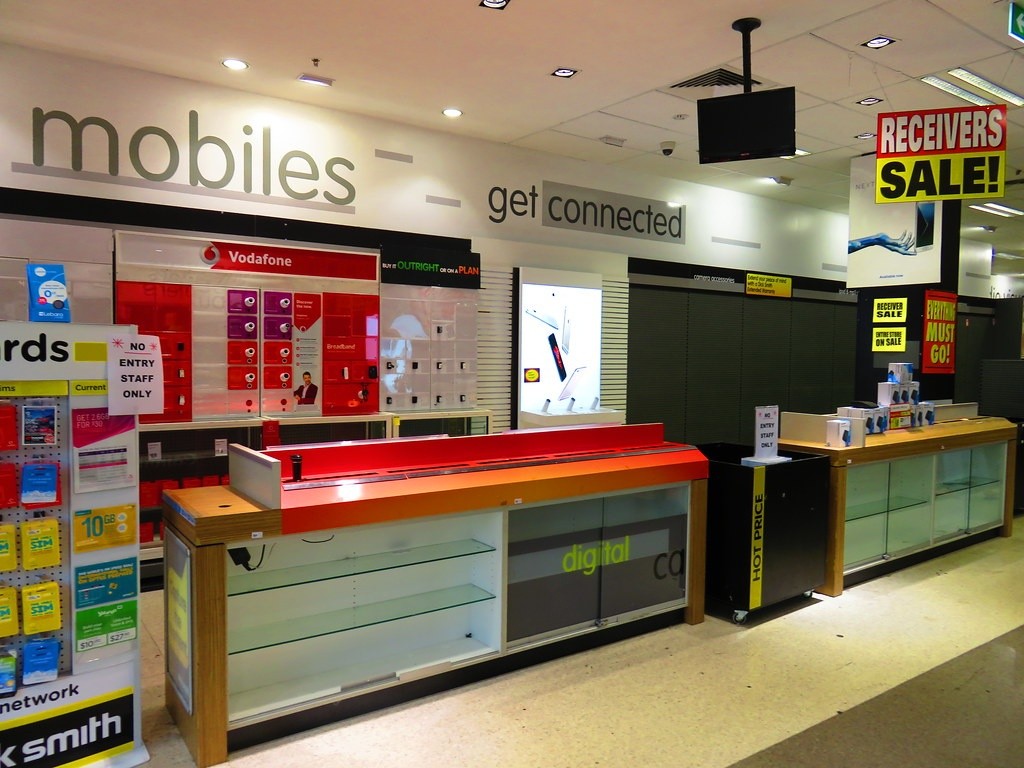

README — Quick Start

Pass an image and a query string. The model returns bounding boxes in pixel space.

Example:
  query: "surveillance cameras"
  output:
[660,141,676,156]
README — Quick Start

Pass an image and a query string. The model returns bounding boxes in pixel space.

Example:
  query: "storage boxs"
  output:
[837,362,935,435]
[826,420,851,447]
[698,442,830,626]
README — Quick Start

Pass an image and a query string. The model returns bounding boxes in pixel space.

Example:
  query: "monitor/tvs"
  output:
[698,86,796,165]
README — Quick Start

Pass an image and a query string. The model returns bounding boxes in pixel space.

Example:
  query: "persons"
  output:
[293,372,319,405]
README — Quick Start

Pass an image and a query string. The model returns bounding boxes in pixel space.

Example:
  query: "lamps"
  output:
[297,72,336,87]
[549,65,582,81]
[479,0,510,10]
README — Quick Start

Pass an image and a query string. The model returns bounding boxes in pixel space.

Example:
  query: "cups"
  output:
[291,455,302,481]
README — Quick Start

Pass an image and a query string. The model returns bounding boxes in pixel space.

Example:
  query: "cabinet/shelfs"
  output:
[772,415,1017,598]
[159,443,710,768]
[139,405,493,562]
